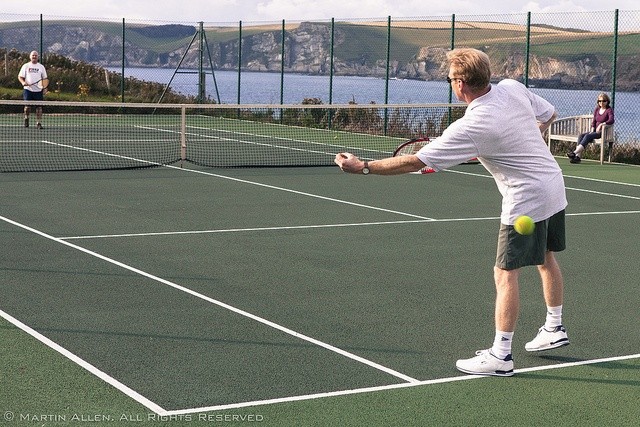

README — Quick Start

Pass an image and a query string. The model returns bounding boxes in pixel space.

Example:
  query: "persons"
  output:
[333,48,571,376]
[567,92,614,163]
[18,51,48,129]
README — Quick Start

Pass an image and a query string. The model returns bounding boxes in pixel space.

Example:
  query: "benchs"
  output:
[547,114,614,166]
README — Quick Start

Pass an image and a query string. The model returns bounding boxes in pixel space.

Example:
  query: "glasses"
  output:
[446,77,463,84]
[598,100,607,103]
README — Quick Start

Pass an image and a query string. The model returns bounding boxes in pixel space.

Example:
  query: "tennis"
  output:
[514,215,535,236]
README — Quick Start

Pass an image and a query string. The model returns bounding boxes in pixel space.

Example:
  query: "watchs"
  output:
[362,160,370,175]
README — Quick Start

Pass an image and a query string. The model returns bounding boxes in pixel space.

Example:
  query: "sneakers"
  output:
[456,349,514,377]
[525,325,570,352]
[570,156,581,164]
[36,123,43,129]
[24,120,29,128]
[568,152,575,158]
